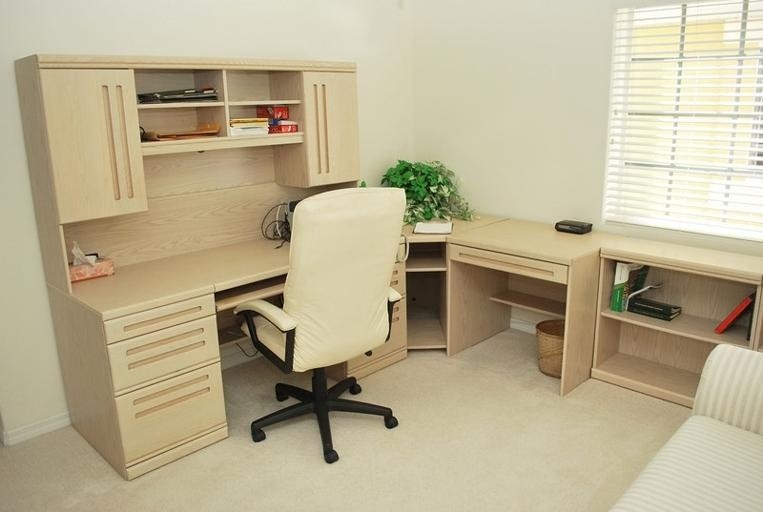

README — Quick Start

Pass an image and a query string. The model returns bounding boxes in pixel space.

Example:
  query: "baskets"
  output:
[536,319,563,379]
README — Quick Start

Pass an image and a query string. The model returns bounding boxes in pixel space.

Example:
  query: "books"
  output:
[625,283,664,311]
[747,301,754,341]
[627,305,680,321]
[713,291,756,334]
[629,298,681,315]
[229,118,270,136]
[609,262,649,312]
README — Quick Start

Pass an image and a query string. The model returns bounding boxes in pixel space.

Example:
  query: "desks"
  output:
[447,219,628,396]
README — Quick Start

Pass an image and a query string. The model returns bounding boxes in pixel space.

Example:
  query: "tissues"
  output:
[67,240,116,283]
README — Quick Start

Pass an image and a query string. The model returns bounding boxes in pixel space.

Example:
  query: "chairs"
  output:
[234,186,407,463]
[607,343,763,512]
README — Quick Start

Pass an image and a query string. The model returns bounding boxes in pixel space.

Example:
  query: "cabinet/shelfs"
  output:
[13,55,149,223]
[47,271,230,481]
[404,236,447,350]
[324,233,407,382]
[271,60,362,188]
[135,54,305,156]
[591,246,763,410]
